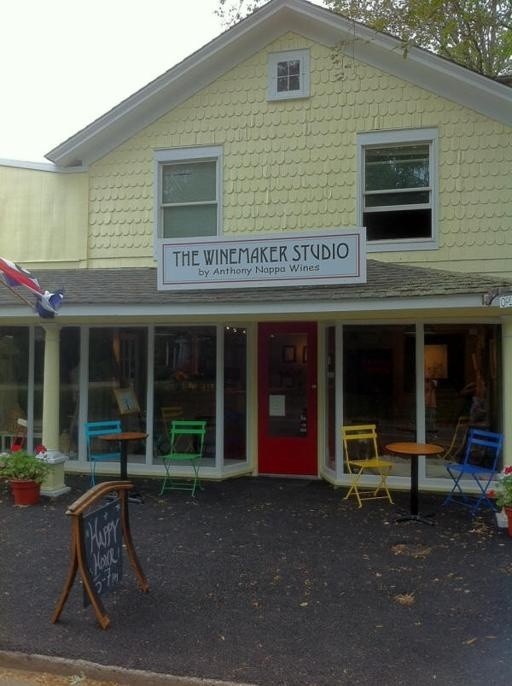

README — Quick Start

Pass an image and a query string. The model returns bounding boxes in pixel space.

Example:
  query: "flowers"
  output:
[488,466,512,508]
[0,443,56,484]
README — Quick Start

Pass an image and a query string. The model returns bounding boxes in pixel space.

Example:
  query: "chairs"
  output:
[159,420,207,498]
[435,416,475,461]
[156,407,196,453]
[440,429,504,522]
[81,420,123,488]
[340,424,393,508]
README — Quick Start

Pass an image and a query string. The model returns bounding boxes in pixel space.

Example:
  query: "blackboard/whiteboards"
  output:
[81,498,122,608]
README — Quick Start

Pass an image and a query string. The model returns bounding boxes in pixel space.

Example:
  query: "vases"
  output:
[504,507,512,540]
[9,480,41,504]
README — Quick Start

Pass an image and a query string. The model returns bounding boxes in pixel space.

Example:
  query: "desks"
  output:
[98,433,148,504]
[384,443,445,527]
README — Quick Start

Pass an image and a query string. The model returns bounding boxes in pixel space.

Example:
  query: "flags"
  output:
[1,256,66,320]
